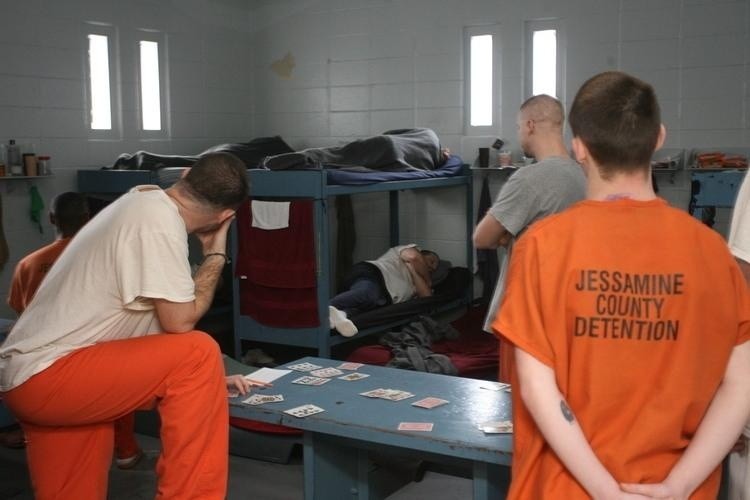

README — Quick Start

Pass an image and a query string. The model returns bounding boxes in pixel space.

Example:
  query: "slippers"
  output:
[117,448,144,470]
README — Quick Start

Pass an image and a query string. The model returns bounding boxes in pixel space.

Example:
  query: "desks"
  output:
[229,356,514,500]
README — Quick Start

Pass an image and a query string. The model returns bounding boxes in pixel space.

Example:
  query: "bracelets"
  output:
[203,253,230,263]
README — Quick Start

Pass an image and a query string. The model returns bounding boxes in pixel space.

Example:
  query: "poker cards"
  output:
[480,382,509,390]
[360,389,416,402]
[413,396,447,408]
[288,362,369,386]
[478,420,514,434]
[243,393,284,406]
[227,386,239,398]
[285,405,322,419]
[398,422,433,432]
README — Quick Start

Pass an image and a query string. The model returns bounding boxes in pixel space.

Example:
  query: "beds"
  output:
[231,163,474,365]
[75,166,185,201]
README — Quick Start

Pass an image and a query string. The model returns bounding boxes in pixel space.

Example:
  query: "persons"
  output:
[472,94,586,334]
[490,72,749,500]
[6,191,143,469]
[329,243,440,337]
[0,150,249,499]
[725,165,749,500]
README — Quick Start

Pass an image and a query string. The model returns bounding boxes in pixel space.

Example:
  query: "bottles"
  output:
[36,156,51,176]
[7,139,20,172]
[0,144,6,176]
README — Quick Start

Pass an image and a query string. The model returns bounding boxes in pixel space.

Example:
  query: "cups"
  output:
[496,152,511,167]
[22,153,38,177]
[479,148,490,166]
